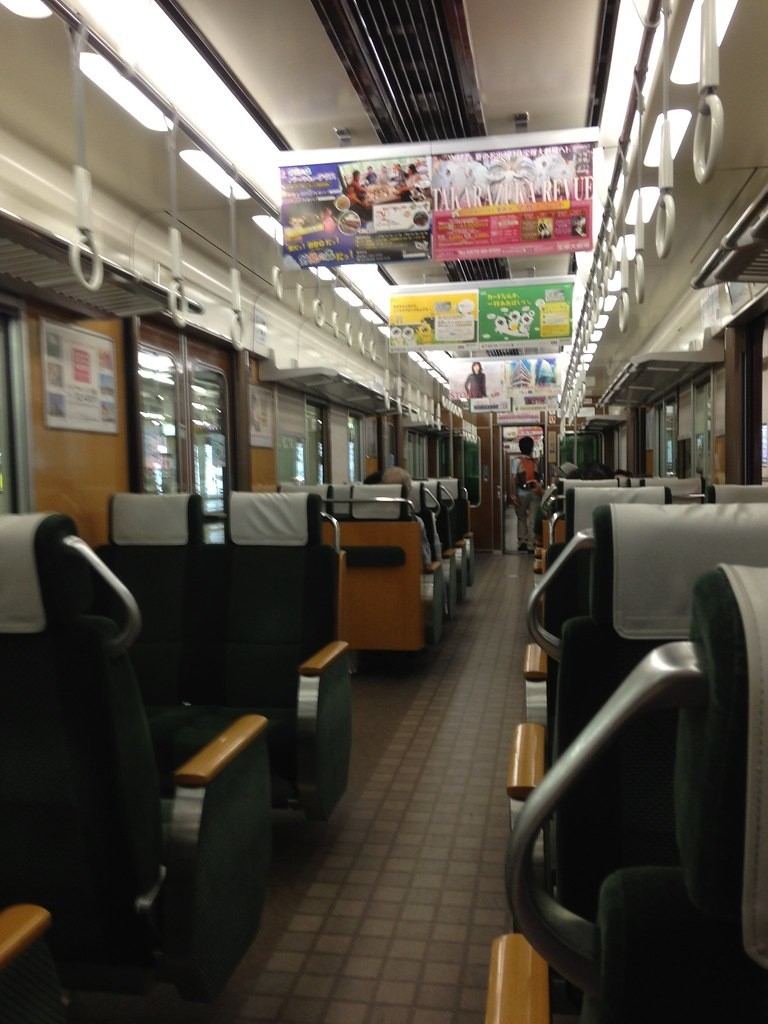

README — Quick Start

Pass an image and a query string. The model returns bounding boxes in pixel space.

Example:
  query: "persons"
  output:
[465,362,488,398]
[511,436,541,553]
[534,460,634,544]
[539,219,549,239]
[346,163,421,208]
[364,466,432,562]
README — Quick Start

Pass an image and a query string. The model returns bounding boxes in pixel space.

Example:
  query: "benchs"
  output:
[0,478,768,1024]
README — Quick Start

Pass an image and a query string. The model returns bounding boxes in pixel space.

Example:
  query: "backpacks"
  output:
[516,456,541,490]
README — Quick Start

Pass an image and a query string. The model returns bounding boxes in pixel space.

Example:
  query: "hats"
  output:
[576,461,614,480]
[551,461,579,479]
